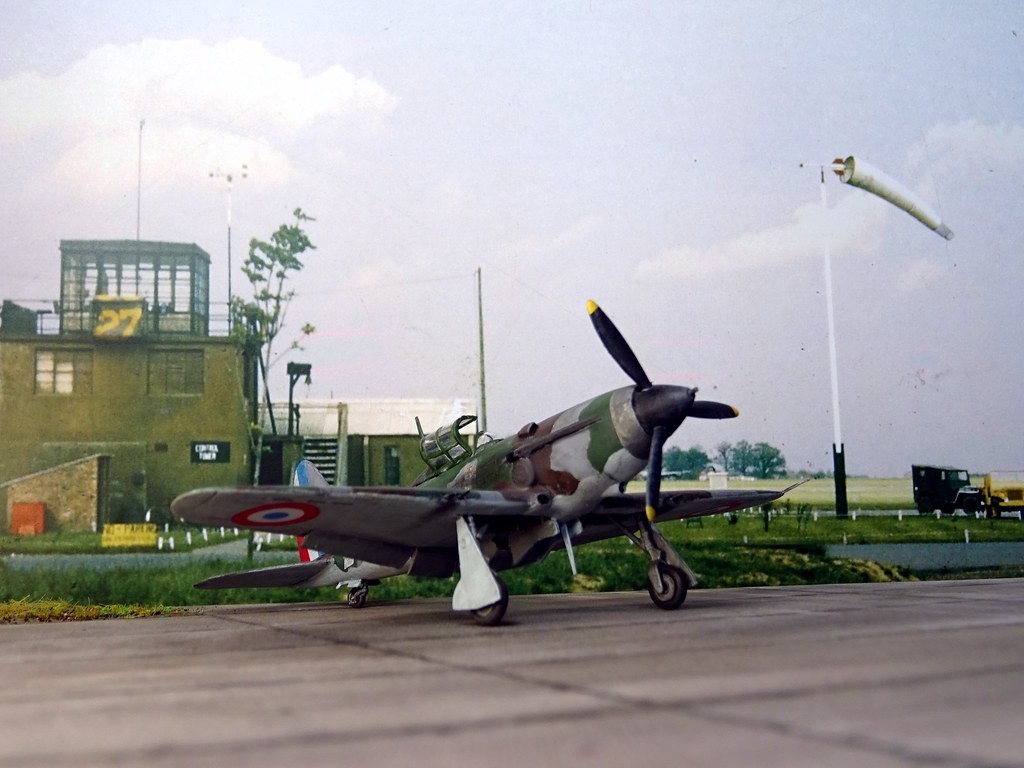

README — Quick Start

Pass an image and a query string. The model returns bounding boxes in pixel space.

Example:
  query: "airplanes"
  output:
[161,293,813,628]
